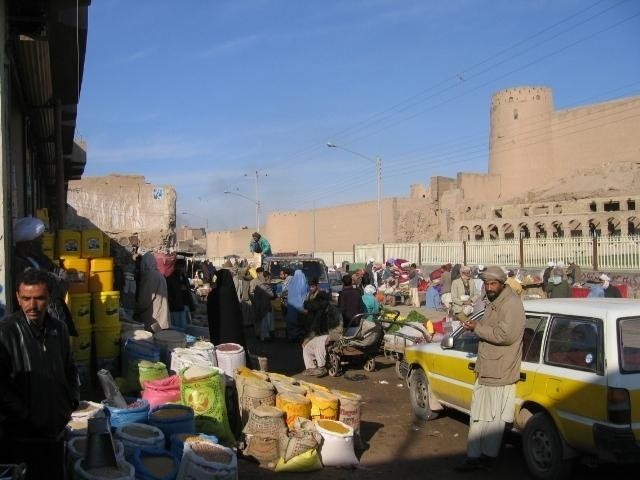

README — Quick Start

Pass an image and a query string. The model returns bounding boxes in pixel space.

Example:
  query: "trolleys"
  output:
[303,310,400,379]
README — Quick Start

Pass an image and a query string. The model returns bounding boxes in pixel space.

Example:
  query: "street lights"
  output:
[325,141,383,245]
[181,211,210,236]
[223,189,261,235]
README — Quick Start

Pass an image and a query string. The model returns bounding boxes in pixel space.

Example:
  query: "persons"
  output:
[12,216,82,393]
[168,232,621,378]
[0,268,82,480]
[460,265,526,470]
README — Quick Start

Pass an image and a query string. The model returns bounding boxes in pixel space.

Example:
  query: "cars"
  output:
[403,297,639,475]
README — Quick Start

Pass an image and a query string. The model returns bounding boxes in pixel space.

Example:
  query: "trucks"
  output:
[257,253,332,339]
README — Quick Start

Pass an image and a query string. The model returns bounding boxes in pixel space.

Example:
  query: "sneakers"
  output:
[456,457,480,470]
[314,367,327,376]
[302,368,316,376]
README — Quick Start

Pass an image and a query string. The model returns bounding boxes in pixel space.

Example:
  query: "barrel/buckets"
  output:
[35,207,122,360]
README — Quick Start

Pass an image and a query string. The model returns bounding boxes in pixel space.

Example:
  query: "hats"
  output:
[307,276,320,285]
